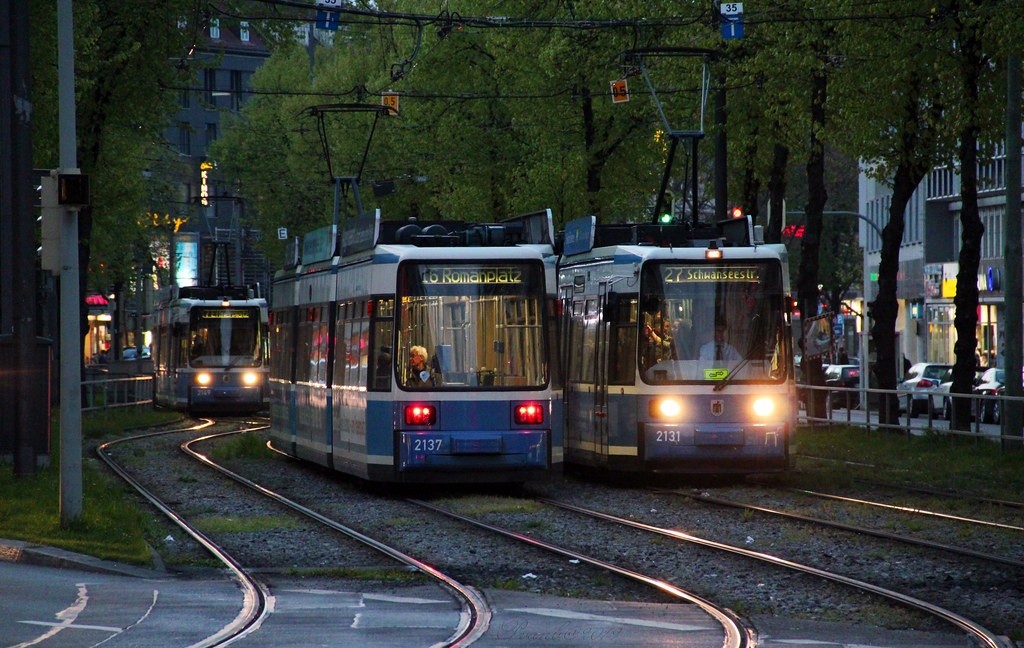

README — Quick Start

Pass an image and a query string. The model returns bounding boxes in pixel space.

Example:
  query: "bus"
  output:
[267,209,565,498]
[151,285,269,413]
[555,208,798,489]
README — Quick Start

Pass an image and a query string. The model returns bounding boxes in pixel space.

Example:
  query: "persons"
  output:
[897,353,912,383]
[982,353,988,367]
[974,339,980,367]
[569,295,692,384]
[700,321,752,377]
[837,347,849,365]
[406,345,443,387]
[192,334,206,357]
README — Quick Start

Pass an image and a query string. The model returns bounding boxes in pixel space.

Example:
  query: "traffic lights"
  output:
[36,176,60,274]
[731,205,744,221]
[658,190,676,225]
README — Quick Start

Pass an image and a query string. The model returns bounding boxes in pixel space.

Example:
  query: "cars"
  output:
[929,365,989,422]
[896,363,953,418]
[824,364,859,409]
[975,367,1024,424]
[89,346,151,364]
